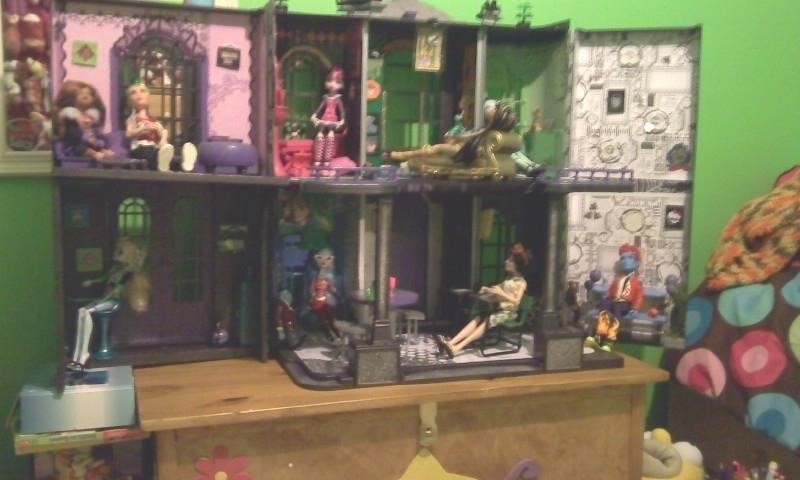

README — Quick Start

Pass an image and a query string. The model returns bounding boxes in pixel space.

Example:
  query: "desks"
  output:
[132,342,670,480]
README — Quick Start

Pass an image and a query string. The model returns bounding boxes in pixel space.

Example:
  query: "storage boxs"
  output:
[22,365,133,433]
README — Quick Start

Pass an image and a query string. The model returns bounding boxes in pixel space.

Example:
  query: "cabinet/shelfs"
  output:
[16,429,151,478]
[54,7,574,371]
